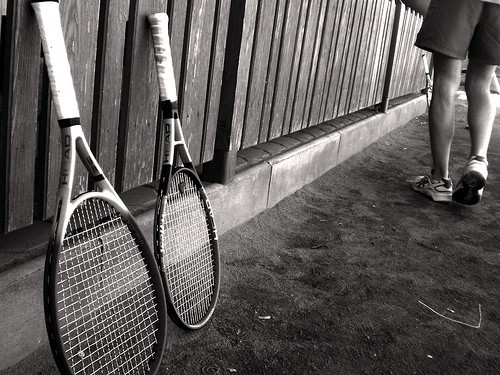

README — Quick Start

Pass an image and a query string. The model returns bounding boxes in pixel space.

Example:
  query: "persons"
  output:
[413,0,500,205]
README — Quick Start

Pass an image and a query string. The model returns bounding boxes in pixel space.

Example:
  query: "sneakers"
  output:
[452,155,488,207]
[412,175,452,203]
